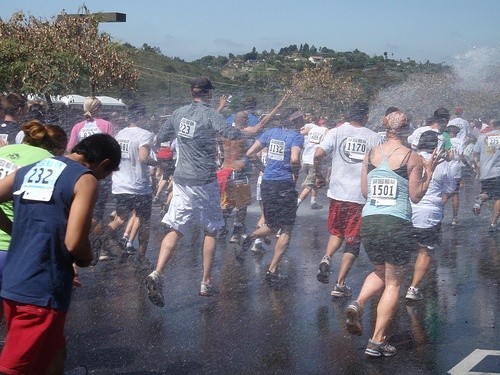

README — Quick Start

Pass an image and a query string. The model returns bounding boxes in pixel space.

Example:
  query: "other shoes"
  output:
[124,247,135,254]
[452,219,463,226]
[473,195,483,216]
[237,235,251,264]
[89,236,102,265]
[72,275,82,287]
[118,239,126,252]
[489,224,500,232]
[266,271,288,286]
[231,234,239,241]
[311,203,323,209]
[99,251,118,260]
[251,244,265,253]
[219,231,228,237]
[258,225,271,246]
[110,210,117,221]
[133,259,153,272]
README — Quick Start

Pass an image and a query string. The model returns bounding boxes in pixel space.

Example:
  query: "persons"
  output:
[345,110,445,357]
[0,132,122,375]
[0,77,499,307]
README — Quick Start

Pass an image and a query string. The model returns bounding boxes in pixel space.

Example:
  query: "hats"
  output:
[190,76,215,94]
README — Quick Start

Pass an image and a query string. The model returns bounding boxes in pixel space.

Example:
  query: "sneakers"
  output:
[145,271,165,307]
[345,302,362,337]
[405,288,424,300]
[200,283,220,295]
[331,284,353,297]
[316,259,330,283]
[365,336,397,357]
